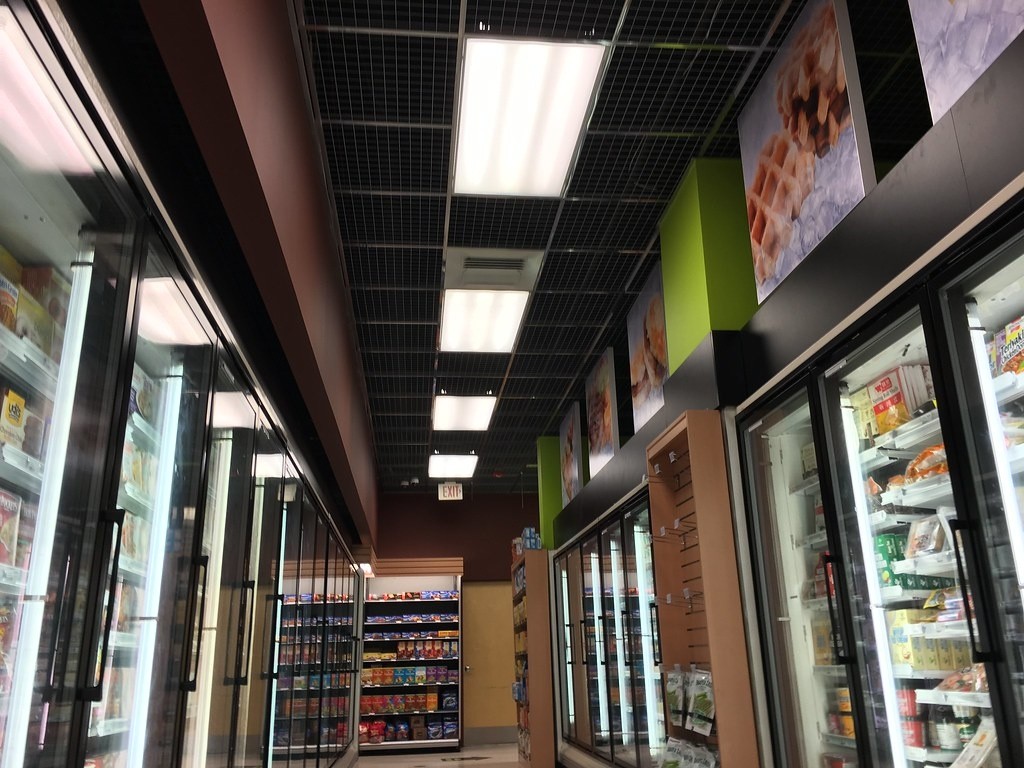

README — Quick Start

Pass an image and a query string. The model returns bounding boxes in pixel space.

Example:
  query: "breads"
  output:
[746,0,853,287]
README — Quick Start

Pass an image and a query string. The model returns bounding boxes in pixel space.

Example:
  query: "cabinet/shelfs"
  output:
[511,549,555,767]
[272,593,354,753]
[359,575,463,749]
